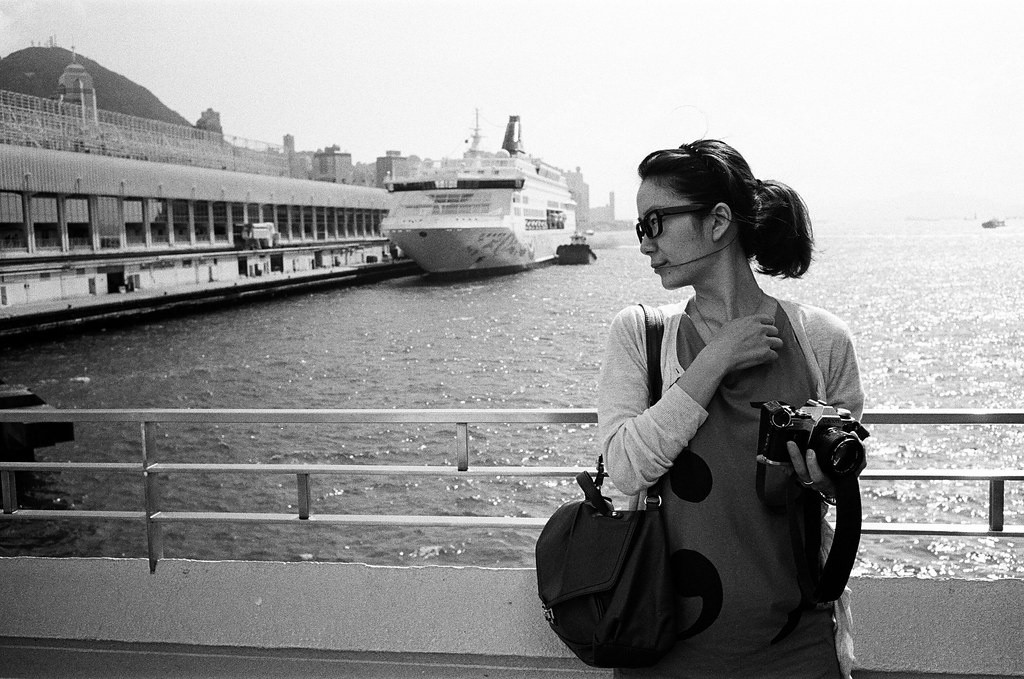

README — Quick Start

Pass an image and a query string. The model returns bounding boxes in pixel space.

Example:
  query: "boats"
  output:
[380,109,576,276]
[0,44,422,344]
[557,231,597,264]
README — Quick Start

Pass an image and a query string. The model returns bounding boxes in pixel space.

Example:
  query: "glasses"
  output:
[636,202,708,245]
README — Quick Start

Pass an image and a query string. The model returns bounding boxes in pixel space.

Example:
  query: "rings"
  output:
[803,480,814,485]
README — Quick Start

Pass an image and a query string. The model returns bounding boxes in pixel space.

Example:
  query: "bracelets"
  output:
[820,486,837,502]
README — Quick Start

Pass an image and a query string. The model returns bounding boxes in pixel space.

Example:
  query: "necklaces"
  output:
[689,286,765,339]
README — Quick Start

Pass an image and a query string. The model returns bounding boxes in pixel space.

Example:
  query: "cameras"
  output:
[756,398,870,483]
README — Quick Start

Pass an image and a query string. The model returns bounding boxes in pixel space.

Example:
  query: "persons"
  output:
[594,137,868,679]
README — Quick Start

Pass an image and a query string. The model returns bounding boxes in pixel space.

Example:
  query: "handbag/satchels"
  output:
[537,472,680,670]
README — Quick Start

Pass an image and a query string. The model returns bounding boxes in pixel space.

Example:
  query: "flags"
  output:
[510,115,519,121]
